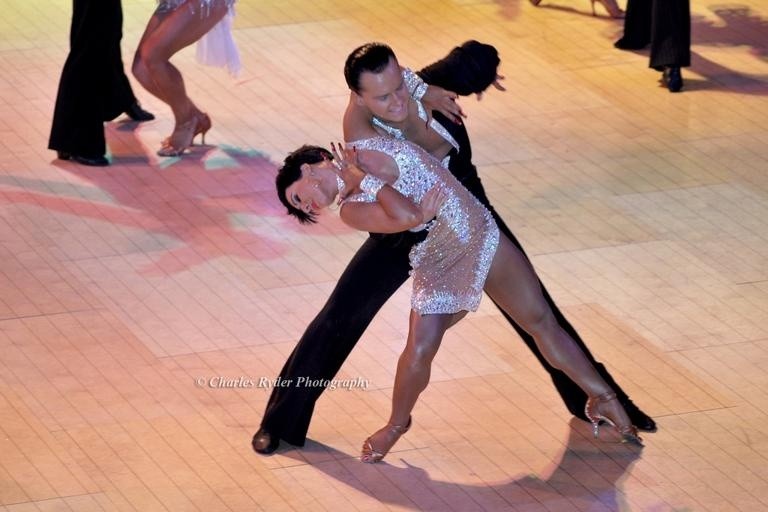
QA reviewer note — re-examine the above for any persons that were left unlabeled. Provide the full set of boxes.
[131,0,239,156]
[251,39,656,453]
[46,0,155,167]
[614,0,691,91]
[529,0,626,18]
[275,66,645,464]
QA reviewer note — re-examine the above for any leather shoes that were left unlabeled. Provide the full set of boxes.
[662,66,684,93]
[252,427,282,454]
[123,102,156,122]
[620,394,656,431]
[57,148,109,167]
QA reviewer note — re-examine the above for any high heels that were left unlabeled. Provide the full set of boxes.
[360,415,413,464]
[584,388,645,448]
[590,0,626,19]
[158,115,213,157]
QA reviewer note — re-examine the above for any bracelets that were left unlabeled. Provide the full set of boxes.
[400,68,429,100]
[359,174,387,200]
